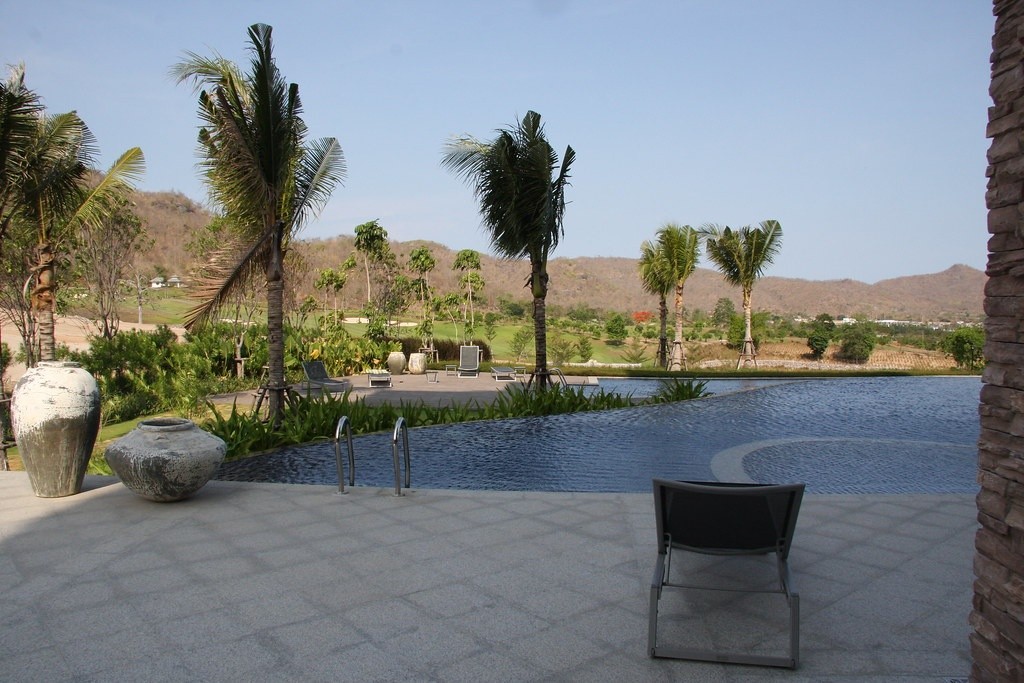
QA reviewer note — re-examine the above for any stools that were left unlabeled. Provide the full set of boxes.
[445,365,457,376]
[514,367,526,377]
[425,370,439,383]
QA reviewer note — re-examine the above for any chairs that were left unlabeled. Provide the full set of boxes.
[366,370,393,388]
[647,477,805,670]
[457,346,480,379]
[301,361,348,394]
[490,366,517,382]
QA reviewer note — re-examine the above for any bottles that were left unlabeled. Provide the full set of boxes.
[387,352,406,375]
[104,416,228,502]
[408,353,427,374]
[10,361,101,498]
